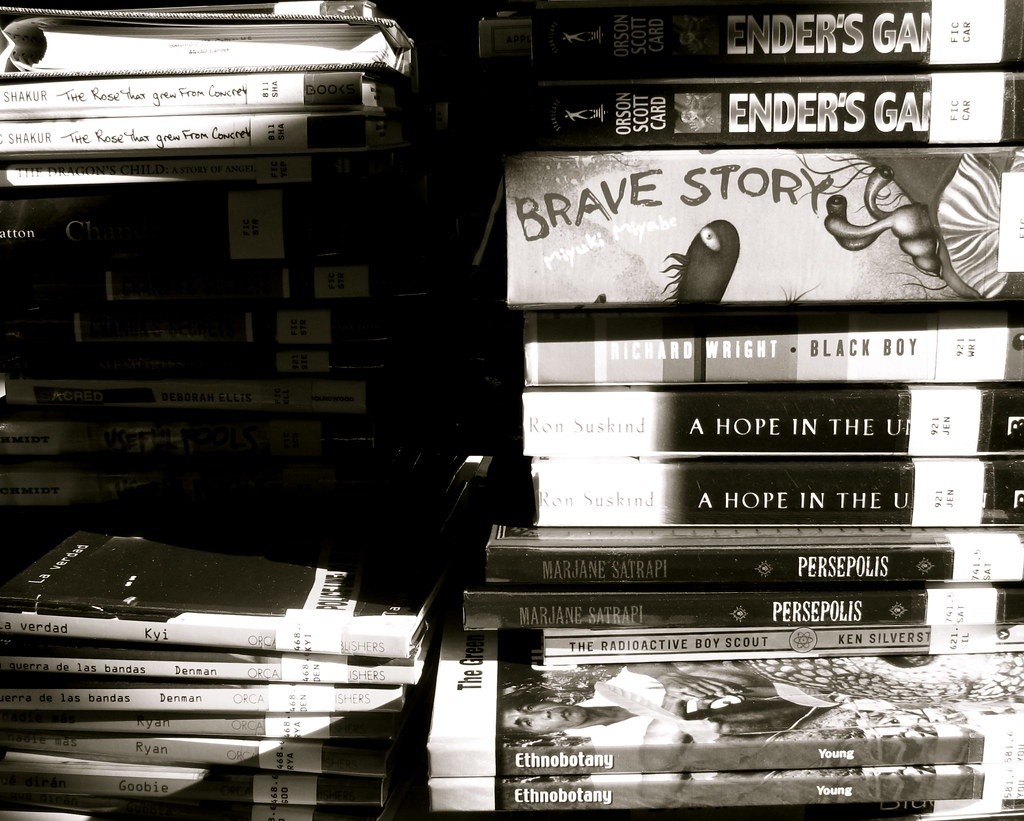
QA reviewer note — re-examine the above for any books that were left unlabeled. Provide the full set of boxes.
[0,0,1023,820]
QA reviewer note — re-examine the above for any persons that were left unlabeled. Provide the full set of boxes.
[499,662,776,767]
